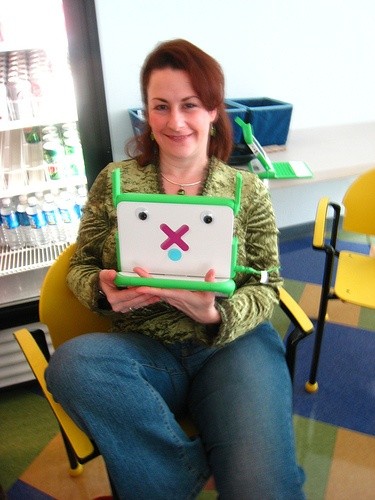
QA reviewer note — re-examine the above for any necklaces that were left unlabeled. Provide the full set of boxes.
[161,173,206,195]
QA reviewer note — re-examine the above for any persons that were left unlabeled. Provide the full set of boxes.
[42,37,309,500]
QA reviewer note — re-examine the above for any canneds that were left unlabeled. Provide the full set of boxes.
[0,50,56,121]
[23,127,40,143]
[40,122,84,179]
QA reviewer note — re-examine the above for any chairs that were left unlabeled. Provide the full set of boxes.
[13,242,315,477]
[305,166,375,394]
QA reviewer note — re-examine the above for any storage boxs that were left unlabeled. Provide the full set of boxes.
[127,99,246,155]
[228,97,293,146]
